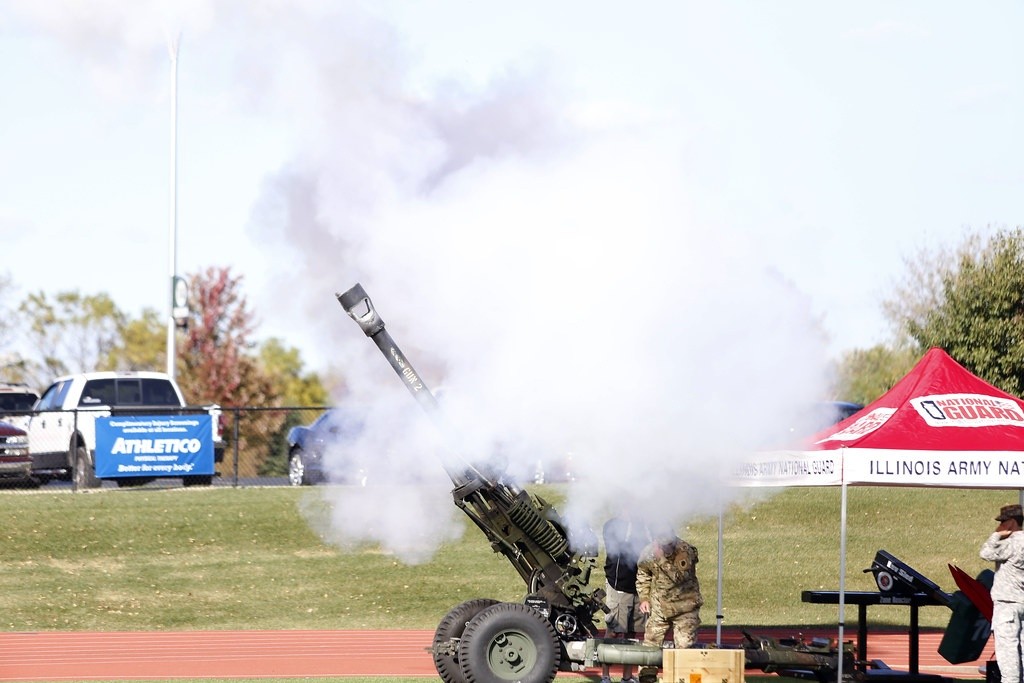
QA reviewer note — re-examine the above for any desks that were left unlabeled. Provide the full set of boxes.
[801,588,953,683]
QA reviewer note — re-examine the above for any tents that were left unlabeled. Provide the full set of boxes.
[718,346,1024,683]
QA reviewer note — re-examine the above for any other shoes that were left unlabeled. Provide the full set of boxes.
[621,675,639,683]
[601,676,611,683]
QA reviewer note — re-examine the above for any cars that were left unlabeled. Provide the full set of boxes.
[1,384,40,417]
[0,421,31,484]
[285,390,378,485]
[804,402,862,429]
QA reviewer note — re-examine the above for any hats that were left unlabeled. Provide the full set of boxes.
[995,504,1023,521]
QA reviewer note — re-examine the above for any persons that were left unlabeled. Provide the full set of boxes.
[635,520,704,683]
[602,499,651,683]
[978,504,1024,683]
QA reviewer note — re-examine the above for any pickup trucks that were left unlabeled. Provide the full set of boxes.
[3,371,227,491]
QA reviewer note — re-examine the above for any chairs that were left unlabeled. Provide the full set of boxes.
[143,383,169,405]
